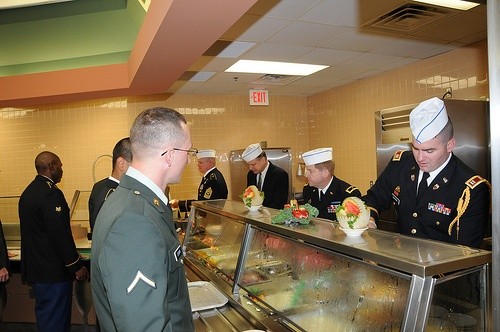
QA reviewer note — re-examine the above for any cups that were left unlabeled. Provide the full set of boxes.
[180,212,185,219]
[172,208,178,220]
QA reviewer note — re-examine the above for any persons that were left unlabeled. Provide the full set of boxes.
[172,149,229,216]
[86,136,131,239]
[300,147,361,222]
[0,219,18,312]
[240,143,290,209]
[89,108,198,332]
[359,97,492,300]
[18,151,86,332]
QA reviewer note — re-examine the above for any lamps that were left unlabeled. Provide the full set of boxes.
[296,162,306,175]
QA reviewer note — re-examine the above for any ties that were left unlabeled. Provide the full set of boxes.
[319,189,325,205]
[415,171,431,206]
[257,172,262,191]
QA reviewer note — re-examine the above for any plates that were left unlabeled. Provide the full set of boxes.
[361,295,407,303]
[423,305,476,331]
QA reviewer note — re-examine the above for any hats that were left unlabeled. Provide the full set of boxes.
[302,147,333,165]
[197,150,216,158]
[409,96,449,145]
[242,143,263,163]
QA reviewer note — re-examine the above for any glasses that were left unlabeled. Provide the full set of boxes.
[160,147,198,158]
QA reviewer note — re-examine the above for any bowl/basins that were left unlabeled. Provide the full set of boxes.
[206,226,221,235]
[339,226,368,237]
[244,205,262,211]
